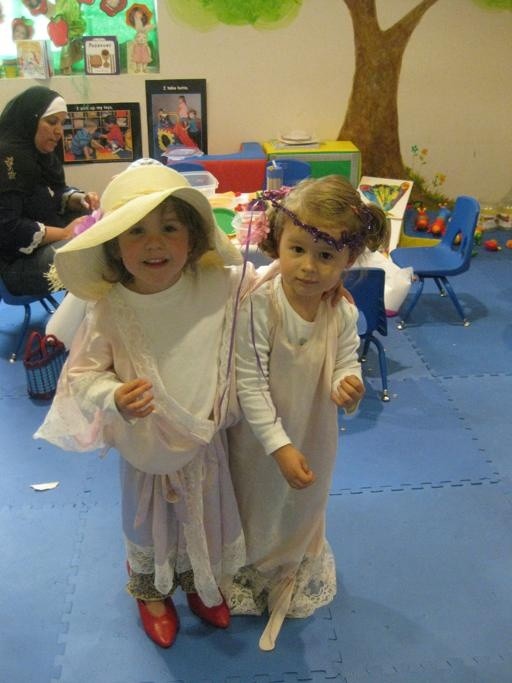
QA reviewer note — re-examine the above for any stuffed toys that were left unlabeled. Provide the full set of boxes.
[414,207,430,232]
[429,201,451,237]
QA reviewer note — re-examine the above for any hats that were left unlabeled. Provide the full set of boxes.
[53,163,245,303]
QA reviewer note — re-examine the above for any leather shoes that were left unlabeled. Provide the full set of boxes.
[137,596,179,648]
[187,586,231,629]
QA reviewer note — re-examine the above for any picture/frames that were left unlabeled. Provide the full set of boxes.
[57,101,140,165]
[82,35,120,76]
[145,77,209,156]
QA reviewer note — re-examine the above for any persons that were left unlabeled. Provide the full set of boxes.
[157,96,201,148]
[228,172,387,650]
[69,115,124,163]
[33,164,353,650]
[1,85,98,299]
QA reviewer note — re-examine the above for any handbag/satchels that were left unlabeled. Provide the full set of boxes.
[24,330,70,399]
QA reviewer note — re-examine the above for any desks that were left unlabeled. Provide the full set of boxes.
[93,181,414,357]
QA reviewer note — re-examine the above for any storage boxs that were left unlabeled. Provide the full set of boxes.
[178,170,219,199]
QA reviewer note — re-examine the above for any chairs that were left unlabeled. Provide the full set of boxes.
[3,282,68,363]
[324,262,394,404]
[389,194,480,329]
